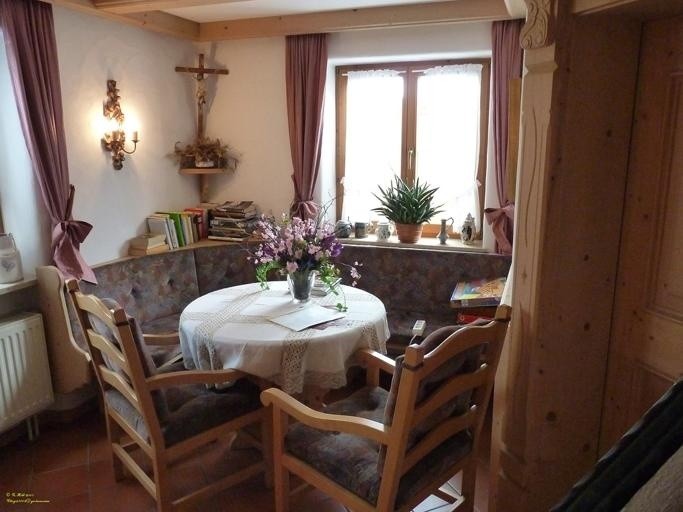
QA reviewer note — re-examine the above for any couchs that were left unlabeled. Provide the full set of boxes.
[63,238,516,377]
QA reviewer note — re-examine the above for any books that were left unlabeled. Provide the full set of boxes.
[128,201,259,257]
[287,274,343,296]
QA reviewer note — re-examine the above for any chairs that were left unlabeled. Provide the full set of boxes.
[258,304,513,512]
[66,278,274,509]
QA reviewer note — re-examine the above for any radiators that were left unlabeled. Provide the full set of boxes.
[0,311,55,440]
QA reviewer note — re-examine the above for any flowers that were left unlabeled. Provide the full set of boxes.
[245,193,365,315]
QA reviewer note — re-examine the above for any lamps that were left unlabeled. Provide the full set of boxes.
[101,118,142,172]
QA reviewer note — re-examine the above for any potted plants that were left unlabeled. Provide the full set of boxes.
[371,174,449,243]
[172,134,237,170]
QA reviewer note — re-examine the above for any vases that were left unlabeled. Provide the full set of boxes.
[284,266,316,303]
